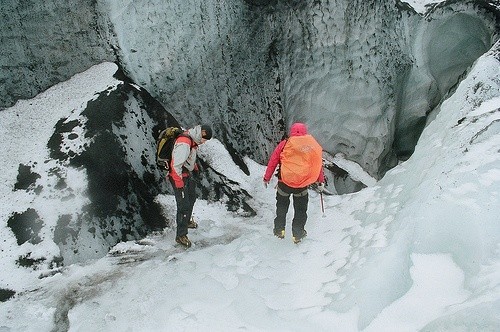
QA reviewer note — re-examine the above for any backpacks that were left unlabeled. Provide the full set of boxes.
[155,124,193,170]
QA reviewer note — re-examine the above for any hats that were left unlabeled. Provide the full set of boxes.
[201,124,212,140]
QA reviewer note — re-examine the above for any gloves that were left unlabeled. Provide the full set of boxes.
[178,187,185,194]
[264,181,270,188]
[317,183,324,192]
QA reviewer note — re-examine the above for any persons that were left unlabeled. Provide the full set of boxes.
[264,122,325,243]
[167,124,212,246]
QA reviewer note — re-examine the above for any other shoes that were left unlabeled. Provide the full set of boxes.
[274,228,285,239]
[293,230,307,244]
[188,221,197,228]
[175,235,191,247]
